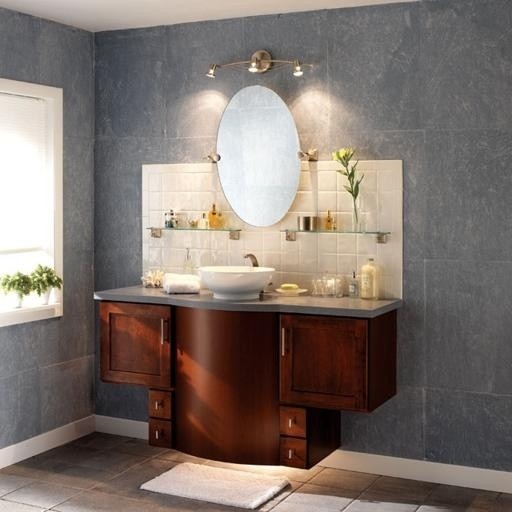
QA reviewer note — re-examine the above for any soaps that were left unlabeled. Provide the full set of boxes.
[280,283,299,291]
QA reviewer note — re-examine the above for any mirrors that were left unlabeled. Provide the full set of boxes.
[214,83,301,228]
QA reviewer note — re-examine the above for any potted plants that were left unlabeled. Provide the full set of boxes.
[28,264,64,308]
[0,269,31,308]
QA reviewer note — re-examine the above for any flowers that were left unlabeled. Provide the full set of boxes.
[332,146,366,224]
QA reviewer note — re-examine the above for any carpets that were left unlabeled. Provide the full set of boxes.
[138,462,289,509]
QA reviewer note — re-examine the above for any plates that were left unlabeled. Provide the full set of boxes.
[275,289,307,293]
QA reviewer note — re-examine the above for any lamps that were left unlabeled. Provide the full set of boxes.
[204,49,305,78]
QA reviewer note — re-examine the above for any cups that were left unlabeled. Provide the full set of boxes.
[312,277,345,296]
[297,216,317,231]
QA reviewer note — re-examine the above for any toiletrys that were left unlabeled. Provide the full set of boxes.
[163,204,226,230]
[360,257,386,300]
[295,209,338,232]
[348,270,358,296]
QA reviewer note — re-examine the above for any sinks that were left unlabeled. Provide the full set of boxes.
[197,265,275,301]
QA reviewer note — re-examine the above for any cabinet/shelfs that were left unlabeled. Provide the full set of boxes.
[147,387,175,450]
[100,302,173,387]
[277,407,343,470]
[280,313,398,415]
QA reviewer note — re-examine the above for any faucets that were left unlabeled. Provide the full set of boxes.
[244,252,258,267]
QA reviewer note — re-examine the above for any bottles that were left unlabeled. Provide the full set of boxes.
[348,272,359,296]
[162,205,228,230]
[324,209,337,230]
[361,258,381,300]
